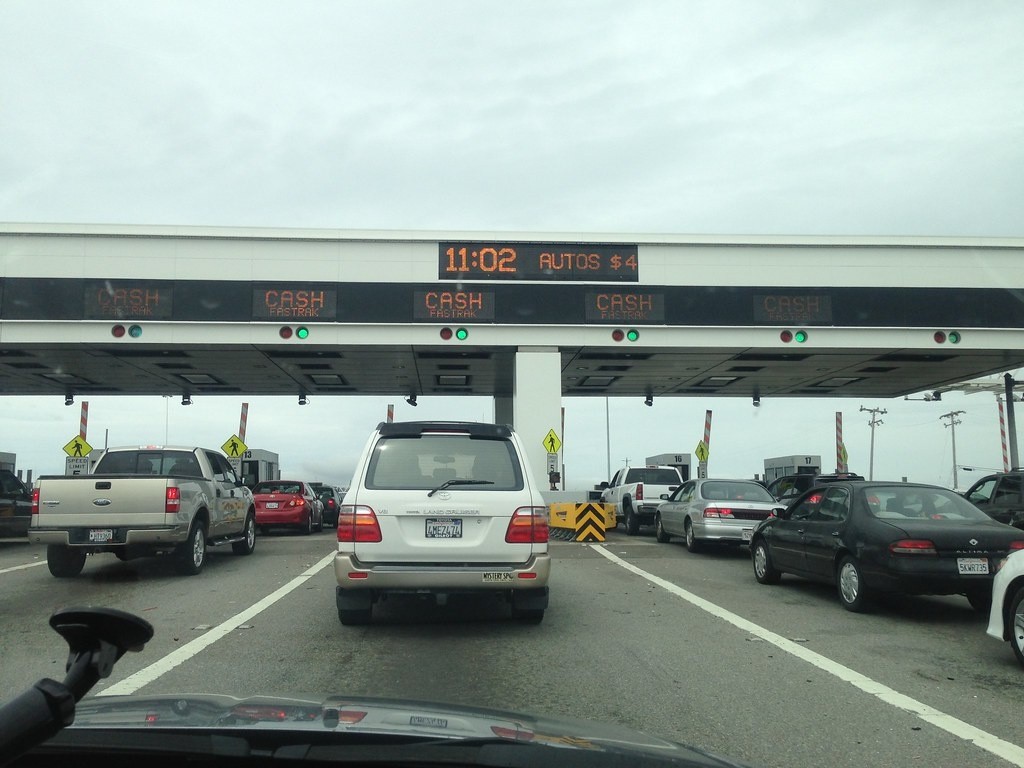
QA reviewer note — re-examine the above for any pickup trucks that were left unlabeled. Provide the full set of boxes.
[26,444,254,577]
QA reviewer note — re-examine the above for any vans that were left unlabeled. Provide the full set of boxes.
[334,419,552,626]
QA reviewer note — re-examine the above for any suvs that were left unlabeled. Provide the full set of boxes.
[598,465,685,536]
[962,465,1024,532]
[767,469,865,520]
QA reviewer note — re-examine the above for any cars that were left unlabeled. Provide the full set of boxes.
[654,476,789,554]
[249,480,325,533]
[747,479,1024,611]
[307,486,340,526]
[986,548,1024,666]
[0,469,31,539]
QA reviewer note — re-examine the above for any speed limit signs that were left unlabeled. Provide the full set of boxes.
[546,453,558,474]
[62,456,89,476]
[698,461,707,480]
[226,457,241,477]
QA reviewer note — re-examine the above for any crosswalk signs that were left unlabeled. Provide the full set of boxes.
[64,436,93,456]
[221,434,248,457]
[695,439,709,463]
[540,428,562,453]
